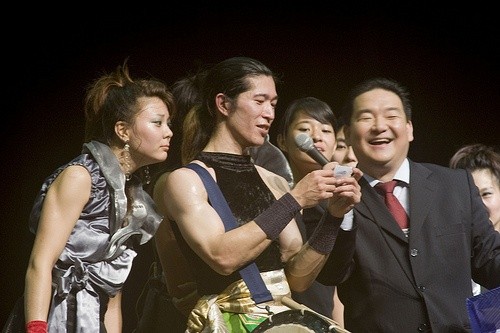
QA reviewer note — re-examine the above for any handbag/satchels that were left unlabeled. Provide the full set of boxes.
[135,262,186,333]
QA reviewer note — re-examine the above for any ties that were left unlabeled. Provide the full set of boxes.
[375,180,411,238]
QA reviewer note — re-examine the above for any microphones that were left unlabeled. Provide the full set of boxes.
[295,133,331,166]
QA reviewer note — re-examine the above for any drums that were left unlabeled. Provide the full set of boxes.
[251,309,351,333]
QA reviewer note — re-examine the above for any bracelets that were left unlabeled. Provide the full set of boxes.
[25,321,48,333]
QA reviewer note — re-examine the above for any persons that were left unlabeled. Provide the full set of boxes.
[152,55,363,333]
[305,77,500,333]
[0,75,174,333]
[138,95,499,333]
